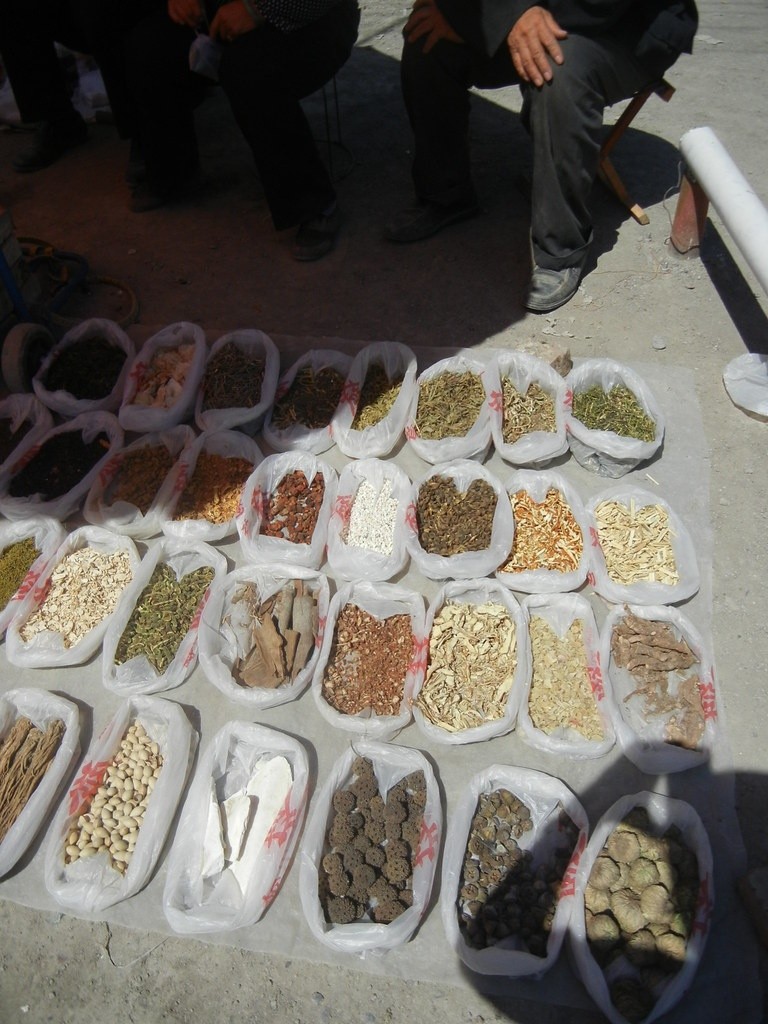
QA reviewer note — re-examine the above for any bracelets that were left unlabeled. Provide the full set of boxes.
[243,0,266,24]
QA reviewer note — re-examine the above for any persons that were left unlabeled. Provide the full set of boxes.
[0,0,361,260]
[378,0,700,311]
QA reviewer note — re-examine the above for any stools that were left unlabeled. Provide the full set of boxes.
[299,53,355,186]
[587,80,676,225]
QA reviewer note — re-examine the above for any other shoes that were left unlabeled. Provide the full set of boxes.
[11,111,88,171]
[128,173,200,211]
[292,206,345,260]
[126,141,159,187]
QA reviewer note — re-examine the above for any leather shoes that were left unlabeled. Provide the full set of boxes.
[521,244,591,311]
[383,192,480,241]
